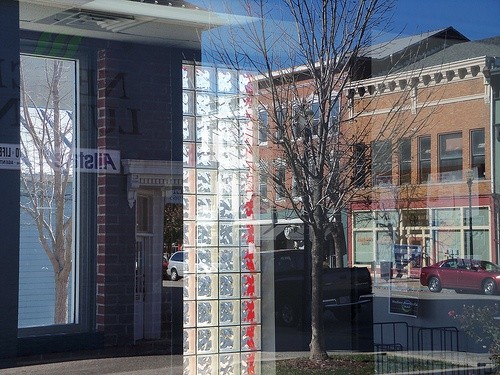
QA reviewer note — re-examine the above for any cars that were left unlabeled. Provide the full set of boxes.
[420,258,500,295]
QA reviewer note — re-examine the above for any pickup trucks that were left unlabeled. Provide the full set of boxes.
[257,248,373,327]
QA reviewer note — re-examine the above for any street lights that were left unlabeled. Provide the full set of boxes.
[465,167,475,259]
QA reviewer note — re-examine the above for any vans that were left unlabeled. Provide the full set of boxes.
[166,250,230,281]
